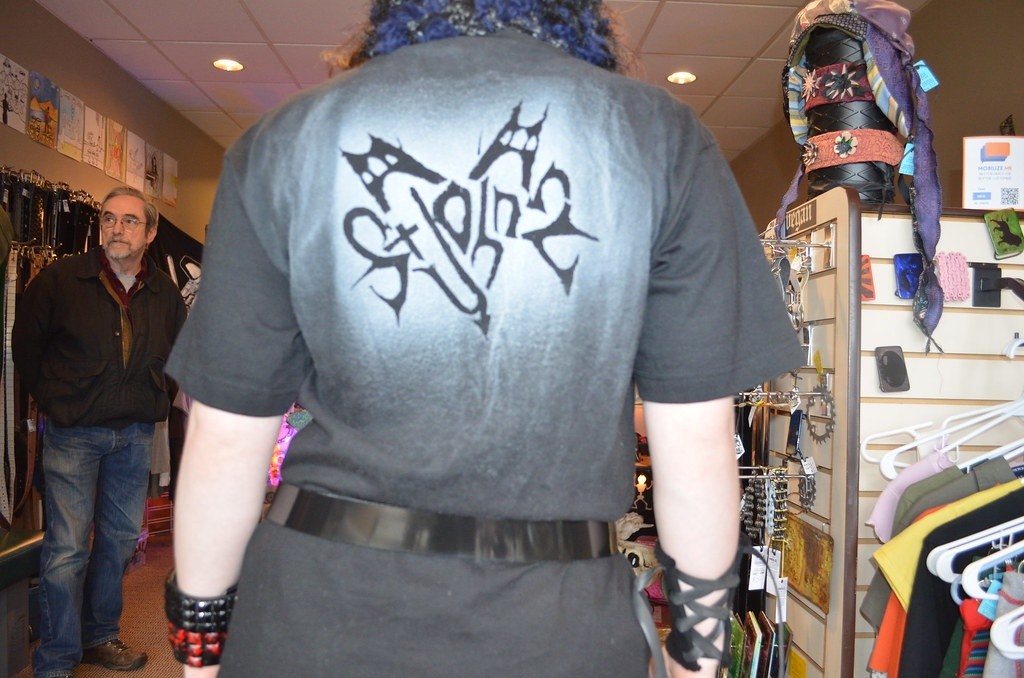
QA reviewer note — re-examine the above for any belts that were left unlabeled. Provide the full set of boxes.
[0,164,104,533]
[268,478,619,562]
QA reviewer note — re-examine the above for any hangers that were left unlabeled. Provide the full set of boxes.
[859,336,1024,661]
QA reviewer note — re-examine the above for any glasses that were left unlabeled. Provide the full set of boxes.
[98,214,151,232]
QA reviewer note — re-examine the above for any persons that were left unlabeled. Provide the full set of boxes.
[162,0,807,678]
[12,187,188,678]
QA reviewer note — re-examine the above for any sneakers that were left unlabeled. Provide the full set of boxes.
[81,640,149,670]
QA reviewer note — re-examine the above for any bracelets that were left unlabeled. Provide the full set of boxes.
[163,568,238,667]
